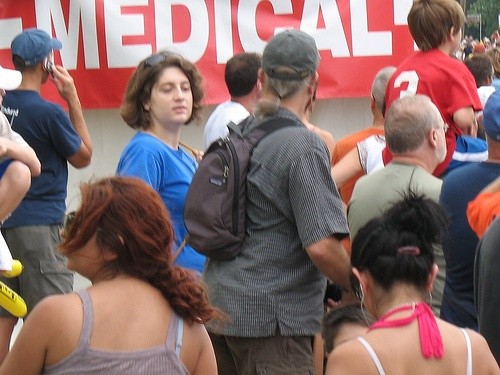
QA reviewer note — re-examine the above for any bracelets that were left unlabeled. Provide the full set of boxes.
[342,287,353,294]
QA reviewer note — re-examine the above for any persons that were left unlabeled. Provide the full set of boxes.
[199,29,361,375]
[0,0,500,375]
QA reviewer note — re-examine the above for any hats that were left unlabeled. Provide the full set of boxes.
[483,90,500,142]
[0,64,22,91]
[10,29,62,71]
[262,29,320,80]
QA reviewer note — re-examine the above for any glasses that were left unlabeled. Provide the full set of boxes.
[143,53,167,71]
[64,213,76,233]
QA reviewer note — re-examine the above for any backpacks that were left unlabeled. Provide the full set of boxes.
[183,117,303,261]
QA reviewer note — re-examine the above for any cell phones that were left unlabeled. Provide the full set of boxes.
[46,61,54,73]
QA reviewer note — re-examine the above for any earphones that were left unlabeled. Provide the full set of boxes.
[360,283,363,293]
